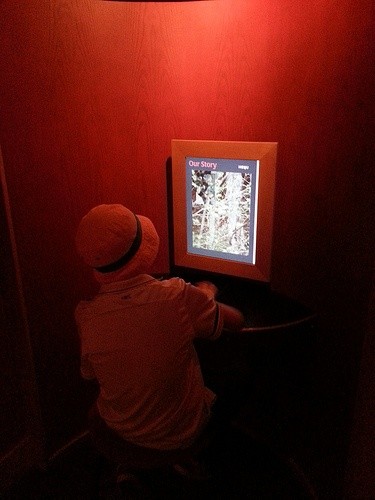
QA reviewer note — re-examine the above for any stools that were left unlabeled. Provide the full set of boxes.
[78,399,204,500]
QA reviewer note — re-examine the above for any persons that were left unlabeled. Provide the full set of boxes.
[75,204,242,449]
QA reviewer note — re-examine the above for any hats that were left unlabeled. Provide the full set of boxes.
[76,204,160,285]
